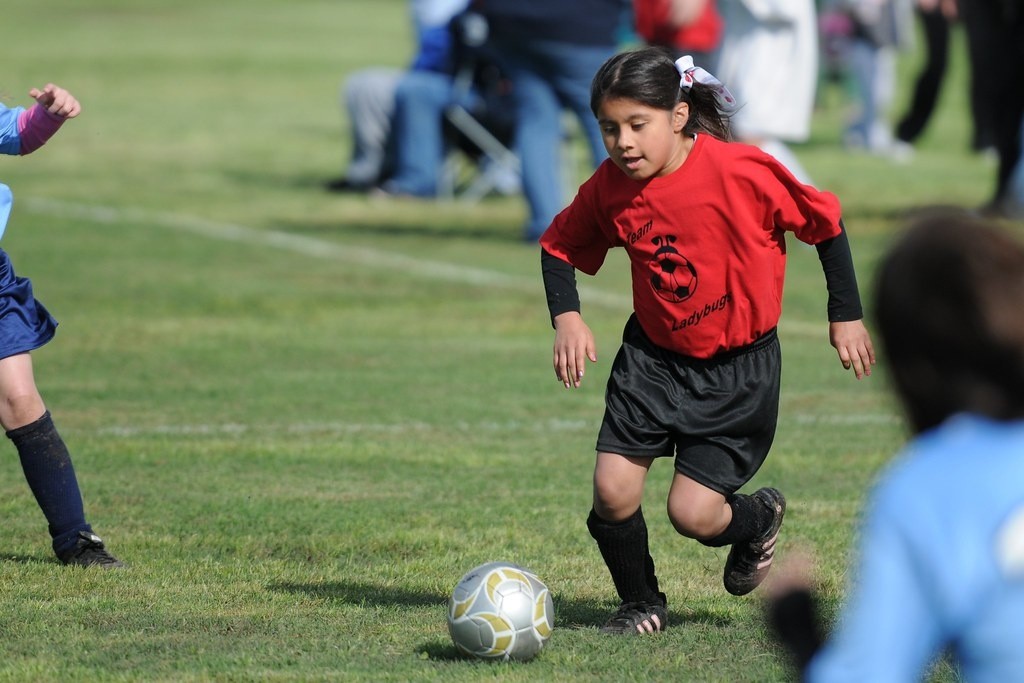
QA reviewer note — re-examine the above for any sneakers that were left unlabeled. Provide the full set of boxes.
[598,598,666,637]
[723,487,786,597]
[54,528,124,572]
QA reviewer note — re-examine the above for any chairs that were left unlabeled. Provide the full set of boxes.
[435,100,523,218]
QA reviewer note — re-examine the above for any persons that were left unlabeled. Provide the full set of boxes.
[540,48,876,636]
[335,0,1024,242]
[0,85,121,566]
[765,207,1024,683]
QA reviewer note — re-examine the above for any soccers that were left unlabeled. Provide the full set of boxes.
[444,558,555,665]
